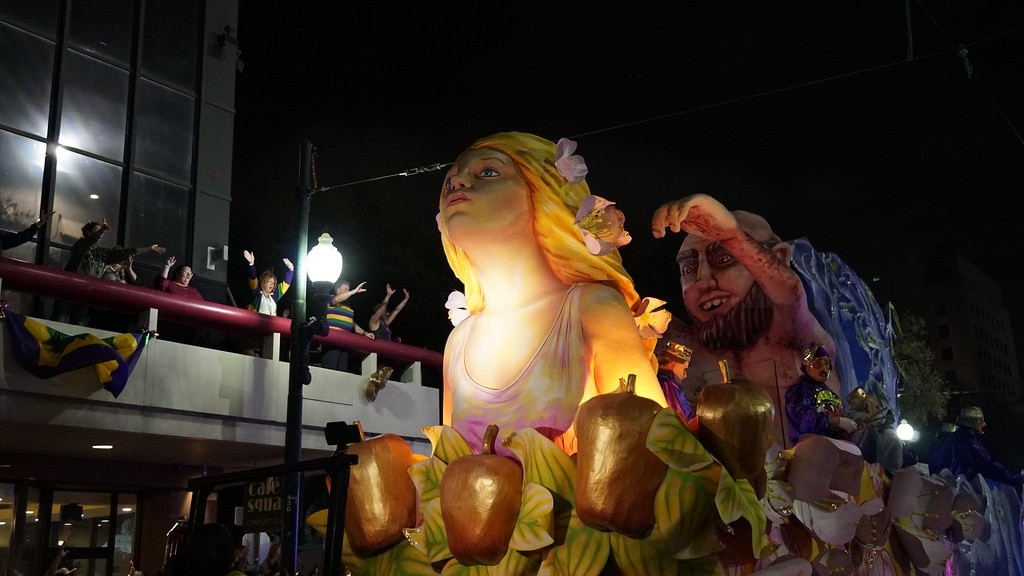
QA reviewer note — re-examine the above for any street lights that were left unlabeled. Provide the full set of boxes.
[283,228,345,576]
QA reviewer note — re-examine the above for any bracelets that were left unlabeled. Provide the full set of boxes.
[361,329,365,335]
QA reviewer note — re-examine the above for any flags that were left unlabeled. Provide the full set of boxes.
[4,309,150,399]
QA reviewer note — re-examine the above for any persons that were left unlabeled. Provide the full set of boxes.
[436,131,669,458]
[369,282,409,380]
[323,279,375,371]
[0,209,56,251]
[653,339,694,421]
[183,522,248,576]
[783,342,867,446]
[651,193,841,453]
[935,405,1024,486]
[243,250,294,358]
[260,544,284,576]
[54,217,210,346]
